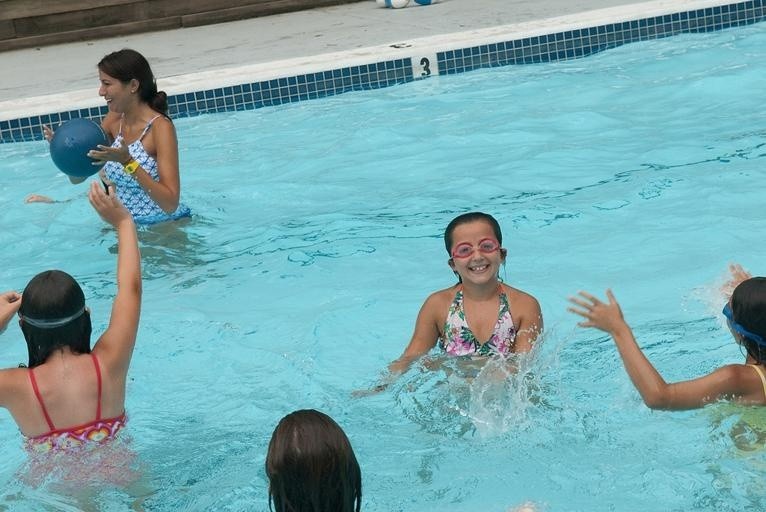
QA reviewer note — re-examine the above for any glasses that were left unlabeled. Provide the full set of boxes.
[722,303,765,347]
[453,238,499,259]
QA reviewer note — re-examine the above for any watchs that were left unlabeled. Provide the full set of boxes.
[125,160,140,174]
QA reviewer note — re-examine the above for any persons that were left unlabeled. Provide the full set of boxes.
[23,191,88,203]
[1,180,144,494]
[563,260,766,454]
[41,47,192,226]
[347,210,545,399]
[263,407,363,512]
